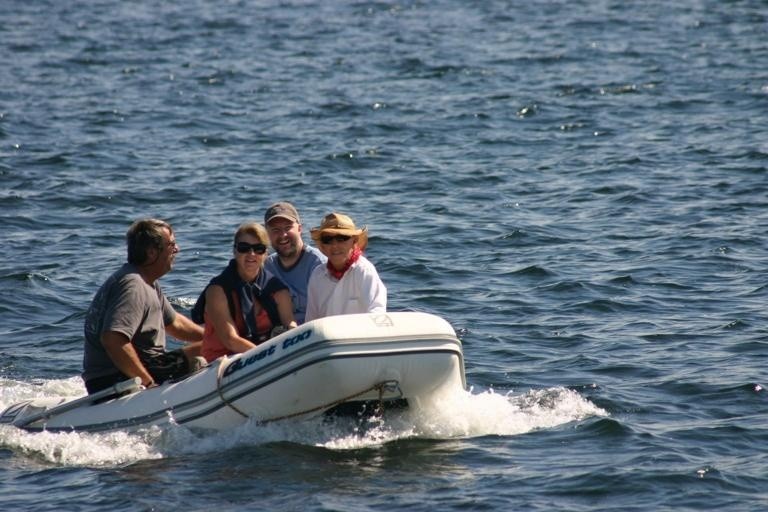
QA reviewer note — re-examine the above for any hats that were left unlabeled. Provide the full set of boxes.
[265,203,299,224]
[312,214,368,256]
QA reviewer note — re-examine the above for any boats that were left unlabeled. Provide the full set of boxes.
[0,312,466,438]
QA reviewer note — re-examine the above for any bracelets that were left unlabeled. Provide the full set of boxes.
[145,380,155,387]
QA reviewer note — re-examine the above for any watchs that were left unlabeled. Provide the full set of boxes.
[301,212,386,322]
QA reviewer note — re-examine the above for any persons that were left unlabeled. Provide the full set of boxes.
[83,219,209,405]
[263,199,327,322]
[202,221,299,362]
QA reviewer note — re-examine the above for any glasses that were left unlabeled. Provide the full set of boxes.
[235,242,267,255]
[320,235,350,244]
